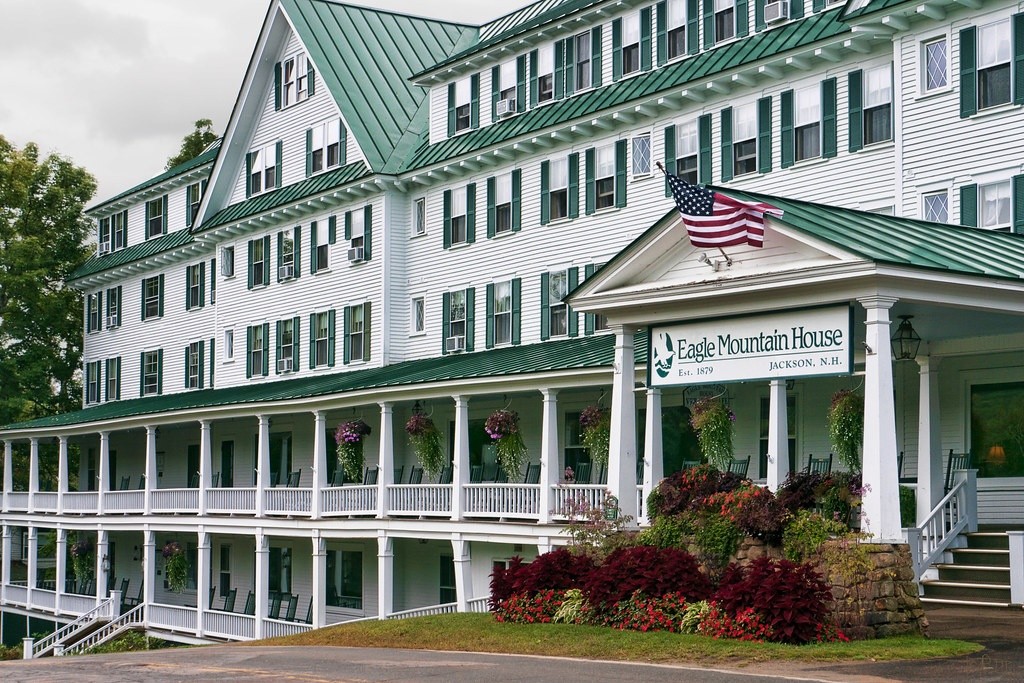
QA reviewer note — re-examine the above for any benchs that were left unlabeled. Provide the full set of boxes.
[35,448,970,624]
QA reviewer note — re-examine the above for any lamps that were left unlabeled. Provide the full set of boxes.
[894,315,920,360]
[419,539,429,544]
[786,380,794,390]
[412,400,423,415]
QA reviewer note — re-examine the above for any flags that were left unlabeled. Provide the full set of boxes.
[666,170,784,248]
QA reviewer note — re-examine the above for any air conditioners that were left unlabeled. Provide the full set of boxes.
[99,241,109,253]
[446,335,465,353]
[279,265,293,279]
[347,247,363,261]
[497,98,513,115]
[105,315,116,327]
[277,358,292,372]
[764,1,787,23]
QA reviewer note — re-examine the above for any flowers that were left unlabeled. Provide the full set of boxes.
[71,541,94,584]
[690,396,736,470]
[407,414,447,480]
[830,389,865,478]
[162,541,190,596]
[583,403,613,466]
[335,417,372,484]
[484,408,531,483]
